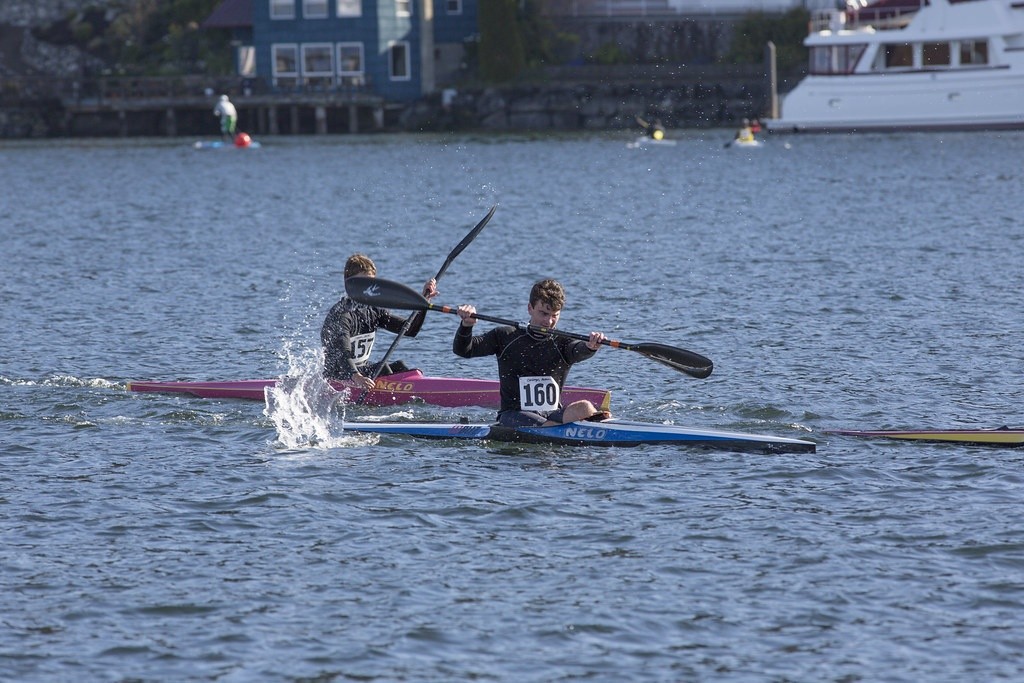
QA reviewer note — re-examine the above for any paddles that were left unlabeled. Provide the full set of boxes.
[633,115,649,130]
[344,276,714,379]
[355,203,499,407]
[723,133,738,148]
[204,88,243,138]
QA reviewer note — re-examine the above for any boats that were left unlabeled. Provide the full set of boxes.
[638,137,676,147]
[843,427,1024,446]
[342,419,820,455]
[765,0,1024,130]
[192,140,260,148]
[727,138,761,149]
[115,369,612,416]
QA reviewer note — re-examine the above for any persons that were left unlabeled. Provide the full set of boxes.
[321,255,439,390]
[735,118,760,142]
[453,279,606,426]
[214,94,237,142]
[646,119,666,139]
